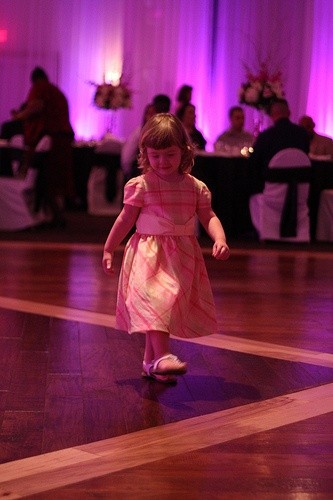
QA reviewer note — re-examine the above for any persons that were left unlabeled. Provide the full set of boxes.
[120,85,332,203]
[102,113,231,386]
[7,67,86,229]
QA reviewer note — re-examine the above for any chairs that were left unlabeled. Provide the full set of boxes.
[0,134,124,232]
[249,148,333,242]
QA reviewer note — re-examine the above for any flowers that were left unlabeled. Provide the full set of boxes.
[86,73,133,109]
[238,40,287,110]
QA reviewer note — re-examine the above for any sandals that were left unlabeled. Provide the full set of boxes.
[141,360,177,383]
[152,353,187,374]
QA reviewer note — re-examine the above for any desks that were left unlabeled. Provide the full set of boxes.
[191,150,330,242]
